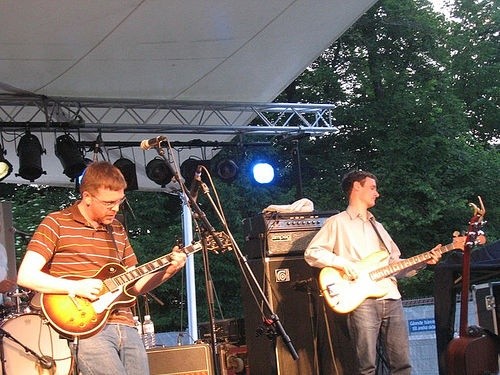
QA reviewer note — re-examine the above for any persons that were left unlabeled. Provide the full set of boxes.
[0,244,15,310]
[15,161,188,375]
[305,171,442,375]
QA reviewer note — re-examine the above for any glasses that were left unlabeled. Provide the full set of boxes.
[92,195,126,207]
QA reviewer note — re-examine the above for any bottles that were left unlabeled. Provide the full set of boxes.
[133,315,143,344]
[143,315,156,350]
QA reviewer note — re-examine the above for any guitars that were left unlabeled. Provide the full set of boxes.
[444,195,500,375]
[318,231,486,315]
[39,231,234,345]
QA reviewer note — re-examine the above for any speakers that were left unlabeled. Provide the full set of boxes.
[145,343,215,375]
[240,256,320,374]
[307,261,364,375]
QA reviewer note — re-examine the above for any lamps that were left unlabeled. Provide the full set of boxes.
[0,121,13,182]
[49,122,92,182]
[179,141,277,188]
[15,121,47,182]
[112,142,138,191]
[143,147,174,188]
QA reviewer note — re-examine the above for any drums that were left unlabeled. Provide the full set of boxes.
[0,312,74,375]
[25,287,41,309]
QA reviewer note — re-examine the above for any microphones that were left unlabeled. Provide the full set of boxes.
[190,166,202,202]
[291,277,314,288]
[140,135,165,150]
[39,355,53,369]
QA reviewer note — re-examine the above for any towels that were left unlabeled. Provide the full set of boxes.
[261,197,315,214]
